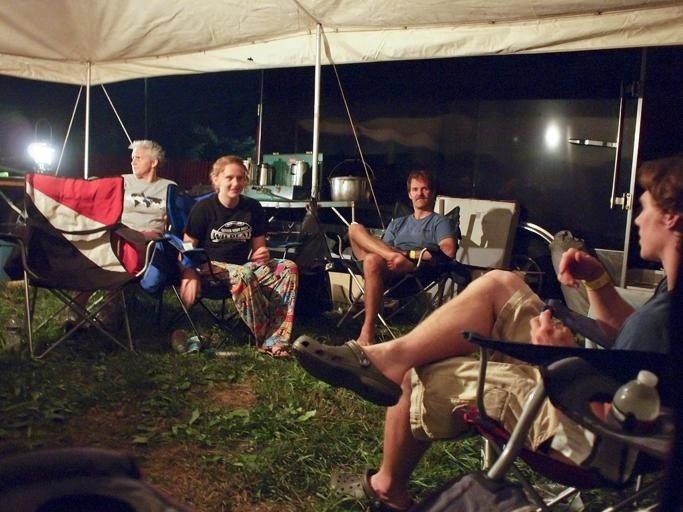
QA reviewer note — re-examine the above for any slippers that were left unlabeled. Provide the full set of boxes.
[292,335,403,409]
[330,467,417,512]
[258,344,290,359]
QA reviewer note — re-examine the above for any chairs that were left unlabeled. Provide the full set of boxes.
[1,172,683,512]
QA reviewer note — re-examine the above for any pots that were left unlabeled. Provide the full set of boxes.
[328,175,376,201]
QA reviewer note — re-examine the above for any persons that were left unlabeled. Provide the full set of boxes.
[59,139,182,344]
[290,154,682,510]
[166,154,300,359]
[346,168,459,345]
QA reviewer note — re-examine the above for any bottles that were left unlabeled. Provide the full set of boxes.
[592,370,660,487]
[171,329,187,353]
[290,160,309,187]
[258,164,275,185]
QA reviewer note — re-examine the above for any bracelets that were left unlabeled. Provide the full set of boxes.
[579,271,611,292]
[408,250,416,260]
